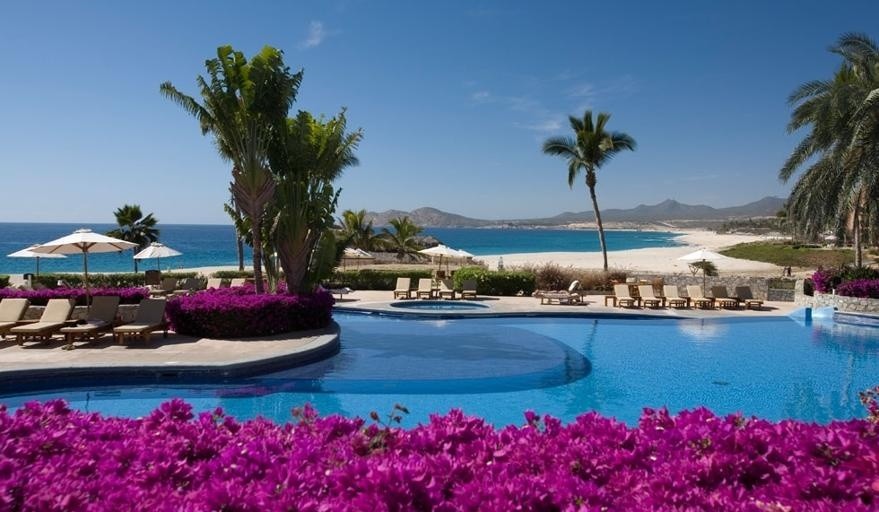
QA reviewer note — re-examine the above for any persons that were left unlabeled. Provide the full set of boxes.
[497,256,502,269]
[178,264,180,269]
[181,265,183,269]
[22,273,35,287]
[785,264,792,276]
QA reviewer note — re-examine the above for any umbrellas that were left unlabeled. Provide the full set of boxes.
[681,249,731,297]
[6,243,68,283]
[26,228,141,313]
[417,243,454,298]
[444,248,466,276]
[456,249,475,267]
[134,242,183,271]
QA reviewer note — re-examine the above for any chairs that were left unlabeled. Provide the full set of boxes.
[532,280,584,305]
[327,286,350,301]
[393,277,411,300]
[613,284,763,310]
[417,278,432,300]
[0,278,248,350]
[439,280,455,300]
[461,279,477,299]
